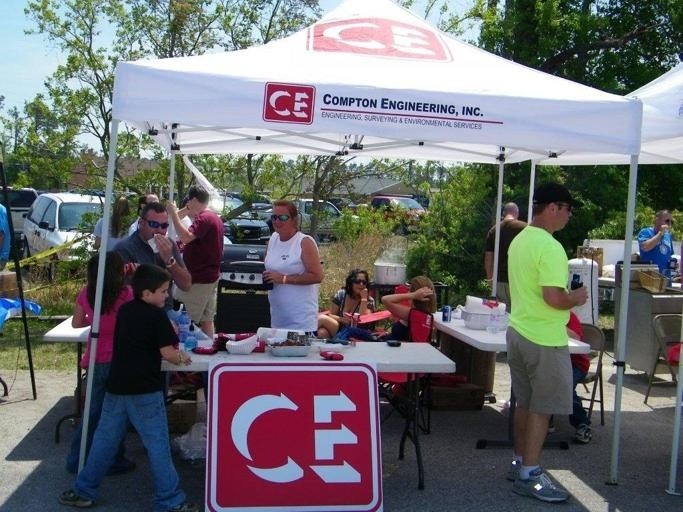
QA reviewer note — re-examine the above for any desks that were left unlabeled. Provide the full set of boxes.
[43,313,91,444]
[161,332,457,488]
[433,316,591,438]
[0,308,22,396]
[597,285,615,315]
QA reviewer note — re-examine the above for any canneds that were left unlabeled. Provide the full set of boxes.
[573,273,580,282]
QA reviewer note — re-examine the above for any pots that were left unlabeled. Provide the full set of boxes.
[373,262,407,286]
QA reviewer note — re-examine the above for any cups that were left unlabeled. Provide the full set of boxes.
[668,258,677,269]
[350,312,361,329]
[262,274,274,291]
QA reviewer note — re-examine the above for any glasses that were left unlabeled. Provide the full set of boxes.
[269,214,291,222]
[142,218,169,230]
[352,278,367,285]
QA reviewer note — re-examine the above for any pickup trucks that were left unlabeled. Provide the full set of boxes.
[225,189,431,242]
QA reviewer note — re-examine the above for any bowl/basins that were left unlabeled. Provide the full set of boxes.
[459,307,511,333]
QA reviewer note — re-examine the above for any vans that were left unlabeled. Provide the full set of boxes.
[1,186,39,239]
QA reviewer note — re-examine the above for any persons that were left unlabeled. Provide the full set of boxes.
[2,183,681,510]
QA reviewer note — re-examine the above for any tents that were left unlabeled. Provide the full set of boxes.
[79,1,683,476]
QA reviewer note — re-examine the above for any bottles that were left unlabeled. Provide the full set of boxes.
[175,304,198,353]
[570,273,583,290]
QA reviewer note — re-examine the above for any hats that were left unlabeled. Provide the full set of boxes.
[531,183,584,207]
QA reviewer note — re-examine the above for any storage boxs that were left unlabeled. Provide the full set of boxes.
[577,245,603,276]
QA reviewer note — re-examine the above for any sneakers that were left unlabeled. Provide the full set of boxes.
[548,414,555,433]
[107,457,136,477]
[506,459,569,503]
[169,500,201,512]
[574,423,592,445]
[56,489,96,508]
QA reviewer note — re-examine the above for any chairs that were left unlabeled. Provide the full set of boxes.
[382,328,443,434]
[164,385,204,405]
[578,323,606,425]
[644,313,683,403]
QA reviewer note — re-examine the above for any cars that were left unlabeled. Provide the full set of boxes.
[179,195,270,244]
[21,191,107,283]
